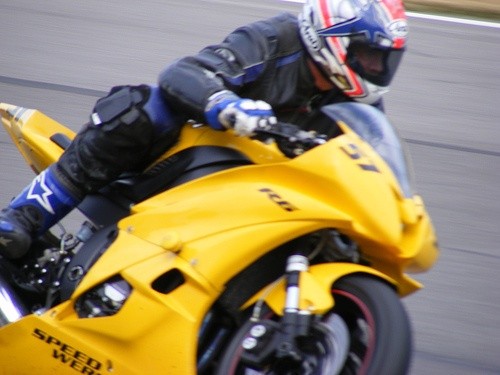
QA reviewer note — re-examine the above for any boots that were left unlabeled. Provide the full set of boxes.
[1,162,87,261]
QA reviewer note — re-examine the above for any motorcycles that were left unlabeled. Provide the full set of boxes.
[0,101,440,375]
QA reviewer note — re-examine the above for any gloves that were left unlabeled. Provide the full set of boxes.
[202,89,277,138]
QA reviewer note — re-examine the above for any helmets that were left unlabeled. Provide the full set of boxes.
[298,0,408,106]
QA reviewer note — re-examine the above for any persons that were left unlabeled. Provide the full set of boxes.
[0,0,408,262]
